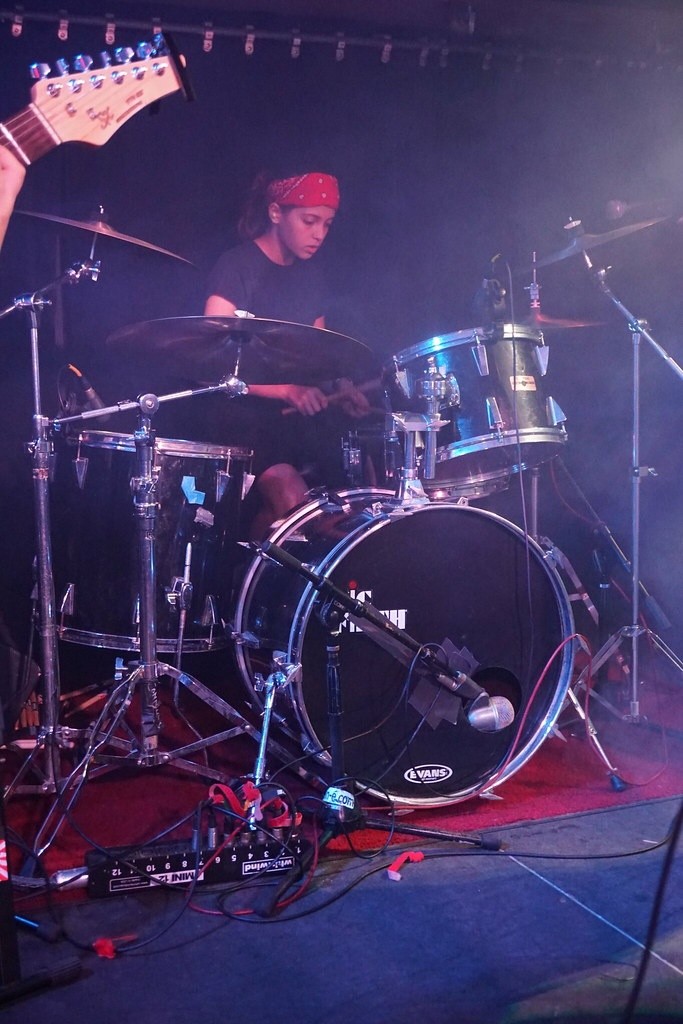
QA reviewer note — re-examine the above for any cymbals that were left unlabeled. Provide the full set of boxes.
[514,306,612,330]
[10,202,201,269]
[100,315,372,391]
[505,213,674,280]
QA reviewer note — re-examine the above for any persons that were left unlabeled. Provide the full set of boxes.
[203,154,371,541]
[0,145,27,251]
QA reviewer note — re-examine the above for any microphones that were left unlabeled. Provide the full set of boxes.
[606,198,667,221]
[480,253,505,313]
[437,675,516,731]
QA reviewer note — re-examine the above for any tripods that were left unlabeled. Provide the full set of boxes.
[0,234,683,917]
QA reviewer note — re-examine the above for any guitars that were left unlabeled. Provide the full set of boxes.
[1,33,192,180]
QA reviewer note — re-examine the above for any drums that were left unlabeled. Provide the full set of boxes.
[38,321,583,808]
